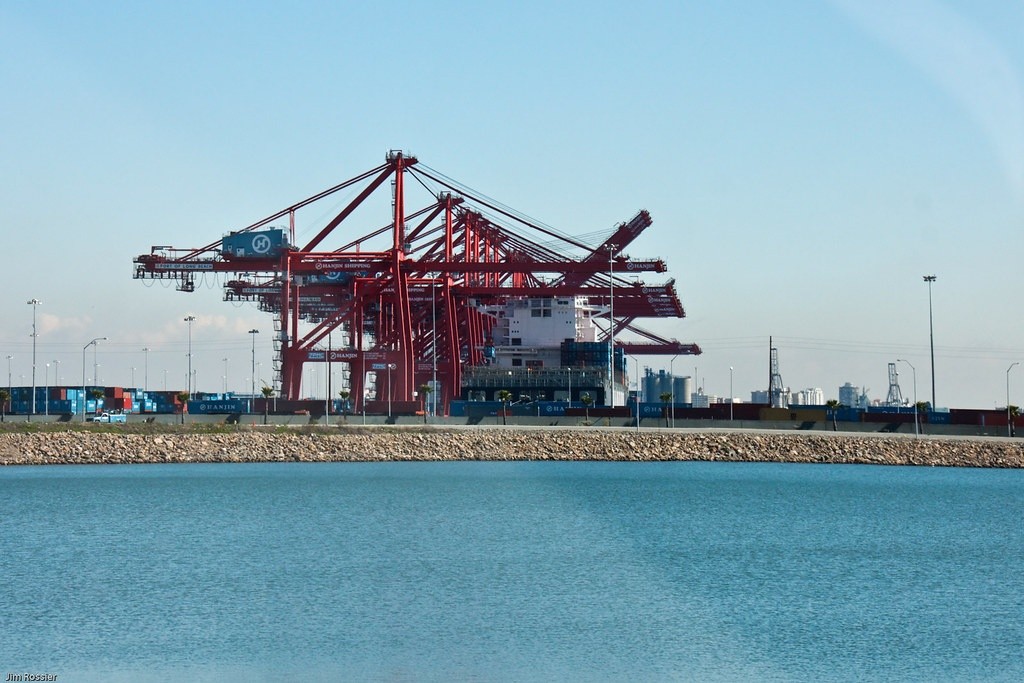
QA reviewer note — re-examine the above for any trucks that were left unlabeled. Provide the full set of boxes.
[85,412,128,425]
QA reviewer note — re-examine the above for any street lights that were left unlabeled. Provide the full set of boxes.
[923,274,937,413]
[670,349,691,429]
[6,353,14,415]
[1006,362,1020,437]
[45,363,50,418]
[567,367,573,409]
[53,359,60,385]
[605,243,621,409]
[81,337,109,423]
[184,314,196,401]
[27,298,42,413]
[729,366,735,423]
[615,350,640,435]
[896,358,920,440]
[248,328,259,423]
[142,347,150,392]
